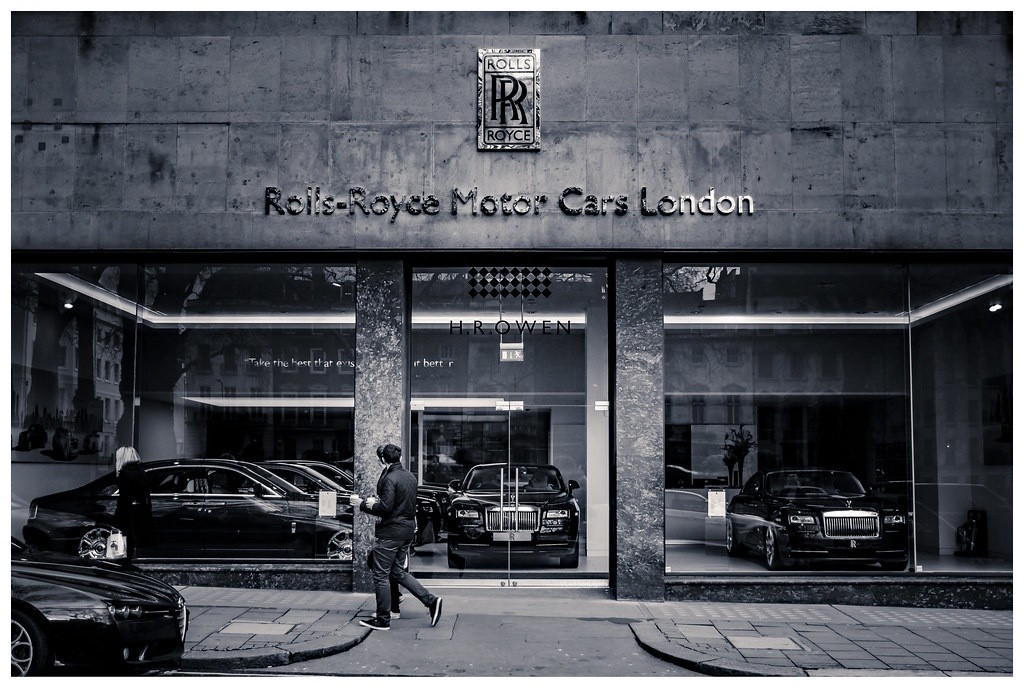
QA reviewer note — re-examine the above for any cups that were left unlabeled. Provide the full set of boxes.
[351,495,359,498]
[367,497,375,503]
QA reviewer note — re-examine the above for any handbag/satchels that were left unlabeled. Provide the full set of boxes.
[106,529,127,560]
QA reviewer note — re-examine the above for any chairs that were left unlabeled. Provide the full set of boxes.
[176,472,197,491]
[524,474,553,491]
[478,474,500,487]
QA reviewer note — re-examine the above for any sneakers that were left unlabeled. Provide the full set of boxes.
[429,597,442,627]
[372,611,400,620]
[359,616,391,630]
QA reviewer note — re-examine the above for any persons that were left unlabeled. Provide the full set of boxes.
[349,443,442,632]
[108,445,155,565]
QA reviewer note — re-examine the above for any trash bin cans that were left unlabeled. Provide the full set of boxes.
[967,510,988,558]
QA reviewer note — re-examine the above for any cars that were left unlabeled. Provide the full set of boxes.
[11,535,191,677]
[726,467,909,570]
[203,462,354,527]
[283,460,443,547]
[444,463,580,569]
[418,485,448,491]
[661,489,727,545]
[416,489,451,528]
[21,459,356,564]
